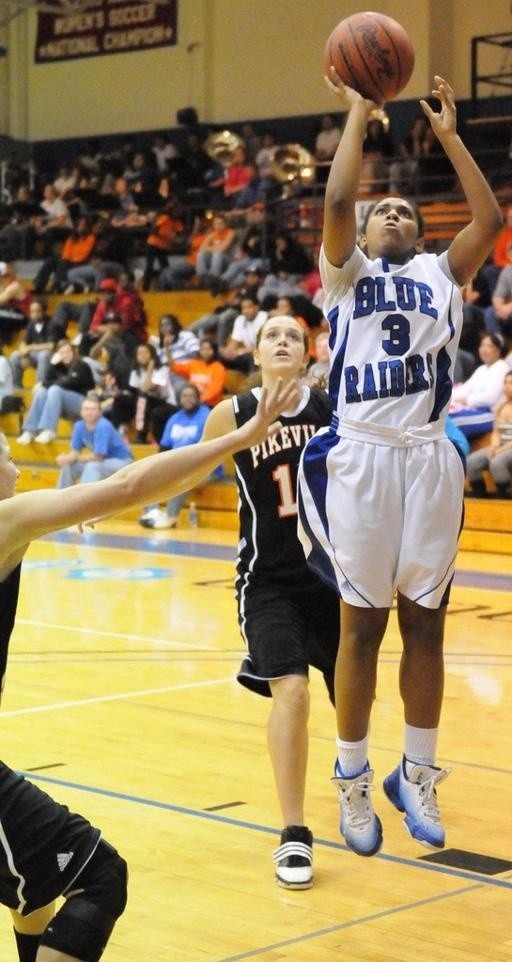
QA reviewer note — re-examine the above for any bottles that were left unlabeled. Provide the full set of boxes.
[190,500,198,530]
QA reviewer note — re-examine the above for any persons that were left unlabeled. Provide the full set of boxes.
[444,333,512,454]
[358,113,453,195]
[57,398,135,489]
[297,65,502,856]
[0,114,343,446]
[0,377,299,961]
[140,386,222,529]
[453,204,512,384]
[198,315,332,890]
[466,372,512,497]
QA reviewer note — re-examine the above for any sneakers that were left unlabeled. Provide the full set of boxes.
[17,432,36,444]
[272,825,313,890]
[334,759,382,856]
[384,752,444,850]
[139,507,177,528]
[36,431,57,443]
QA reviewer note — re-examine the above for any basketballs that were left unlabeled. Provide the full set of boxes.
[324,11,414,103]
